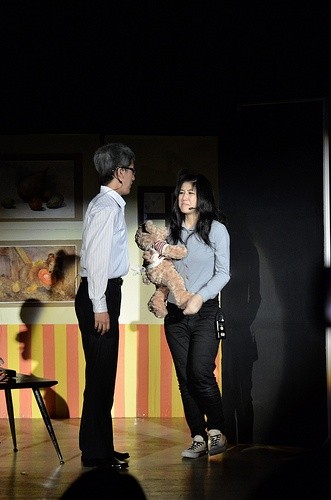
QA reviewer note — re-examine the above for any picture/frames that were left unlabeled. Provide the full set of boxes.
[0,153,83,222]
[0,245,77,304]
[137,186,171,230]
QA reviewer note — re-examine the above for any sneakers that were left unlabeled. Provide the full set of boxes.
[181,435,208,459]
[207,429,229,456]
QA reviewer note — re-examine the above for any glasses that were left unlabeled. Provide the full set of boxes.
[119,167,136,174]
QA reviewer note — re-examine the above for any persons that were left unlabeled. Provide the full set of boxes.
[75,143,135,471]
[164,172,234,459]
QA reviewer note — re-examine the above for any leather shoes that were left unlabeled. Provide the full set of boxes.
[80,450,128,470]
[112,451,130,460]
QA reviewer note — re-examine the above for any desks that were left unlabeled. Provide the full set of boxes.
[0,374,64,465]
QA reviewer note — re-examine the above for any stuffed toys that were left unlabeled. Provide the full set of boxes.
[135,221,194,318]
